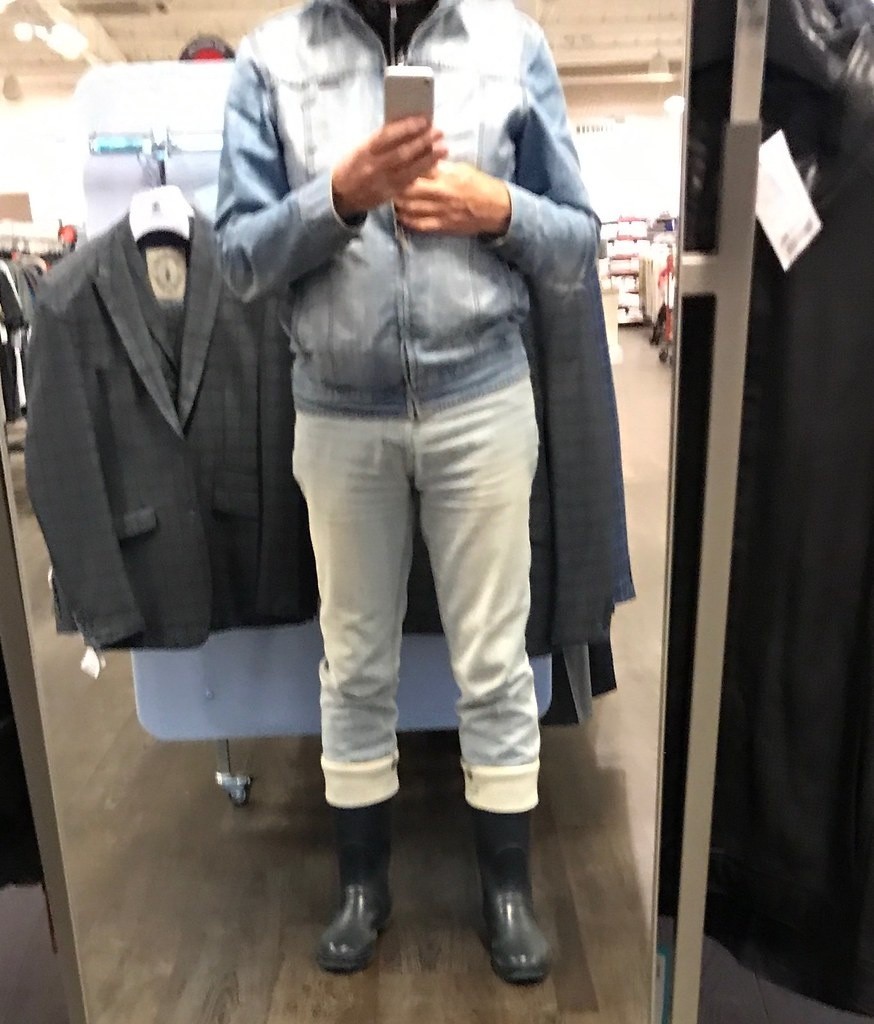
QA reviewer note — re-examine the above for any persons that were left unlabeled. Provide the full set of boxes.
[219,0,597,987]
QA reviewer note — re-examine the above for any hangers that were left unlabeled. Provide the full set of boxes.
[127,136,192,242]
[6,234,33,255]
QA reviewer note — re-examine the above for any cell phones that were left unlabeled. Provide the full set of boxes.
[386,68,433,157]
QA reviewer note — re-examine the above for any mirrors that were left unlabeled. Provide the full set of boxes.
[0,0,779,1024]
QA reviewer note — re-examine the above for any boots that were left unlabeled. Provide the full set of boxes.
[315,799,392,971]
[469,807,551,980]
